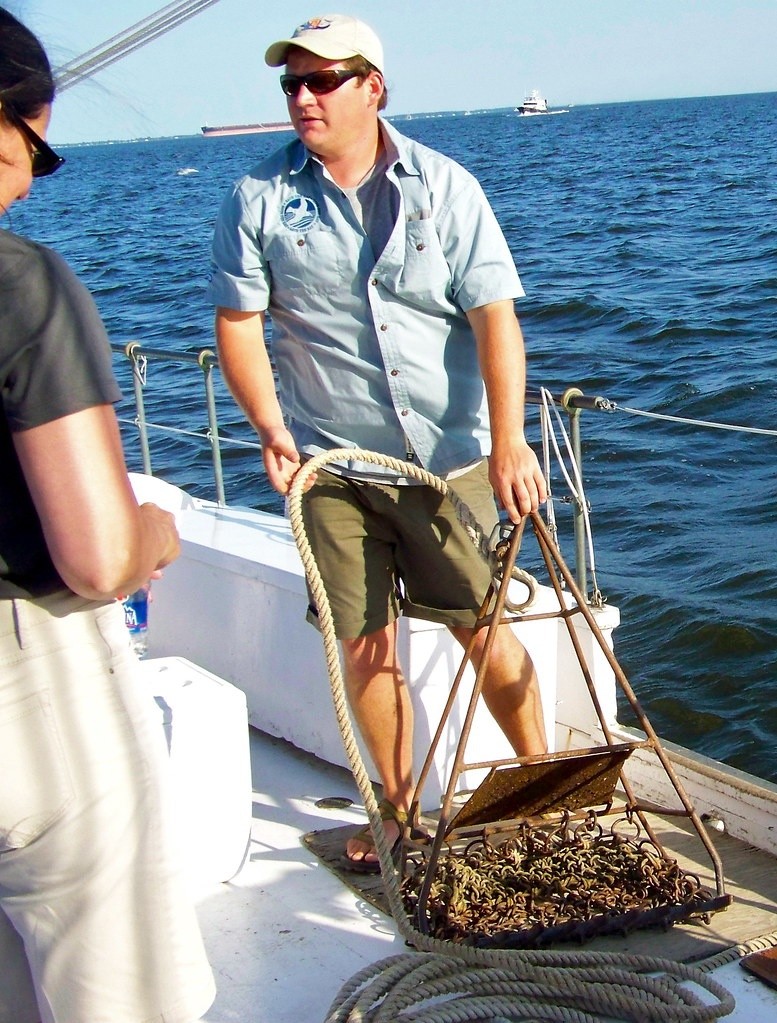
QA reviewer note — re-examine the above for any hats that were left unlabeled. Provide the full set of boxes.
[264,14,383,76]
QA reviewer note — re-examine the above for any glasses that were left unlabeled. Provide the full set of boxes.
[280,70,370,97]
[5,102,65,178]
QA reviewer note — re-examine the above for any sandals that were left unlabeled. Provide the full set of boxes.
[341,798,422,874]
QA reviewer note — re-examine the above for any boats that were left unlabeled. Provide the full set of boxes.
[517,88,549,114]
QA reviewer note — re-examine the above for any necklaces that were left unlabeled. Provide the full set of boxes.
[358,147,388,190]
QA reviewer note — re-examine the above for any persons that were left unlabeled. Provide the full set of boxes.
[210,12,552,867]
[0,7,219,1023]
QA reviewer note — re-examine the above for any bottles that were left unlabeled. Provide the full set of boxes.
[122,588,149,657]
